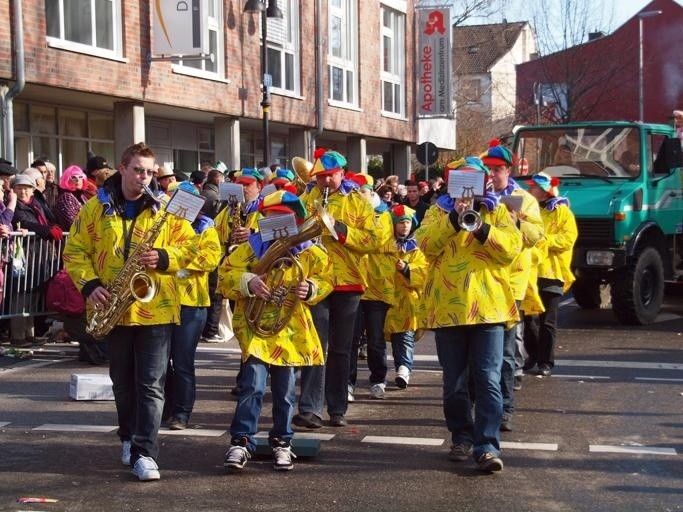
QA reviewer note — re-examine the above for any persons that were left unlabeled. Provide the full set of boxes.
[618,133,641,178]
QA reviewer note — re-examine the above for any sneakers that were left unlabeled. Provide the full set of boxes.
[129,455,161,481]
[447,441,475,462]
[522,355,552,376]
[121,440,132,466]
[268,436,295,471]
[11,336,49,349]
[347,384,356,402]
[358,343,368,358]
[512,375,522,390]
[329,414,347,427]
[368,382,386,400]
[394,364,411,390]
[223,436,252,470]
[469,447,504,472]
[159,415,189,430]
[230,386,242,397]
[292,412,324,429]
[500,411,513,431]
[200,334,225,344]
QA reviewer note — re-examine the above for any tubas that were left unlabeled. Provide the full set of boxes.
[244,198,339,337]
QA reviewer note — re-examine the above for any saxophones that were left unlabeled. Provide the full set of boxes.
[84,183,170,339]
[228,200,243,254]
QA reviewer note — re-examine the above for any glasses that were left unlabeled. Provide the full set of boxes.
[69,175,85,180]
[125,164,154,176]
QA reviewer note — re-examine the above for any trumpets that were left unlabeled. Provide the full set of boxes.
[457,185,484,232]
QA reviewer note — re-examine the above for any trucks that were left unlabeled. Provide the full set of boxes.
[507,119,683,325]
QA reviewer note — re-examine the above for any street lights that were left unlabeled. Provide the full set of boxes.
[240,0,284,167]
[633,5,665,124]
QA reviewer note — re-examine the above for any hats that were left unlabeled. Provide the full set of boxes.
[156,166,177,182]
[443,156,495,195]
[0,157,19,177]
[87,156,114,173]
[258,166,298,199]
[256,190,308,228]
[478,138,520,167]
[524,170,560,198]
[308,148,348,178]
[390,204,419,232]
[11,175,34,189]
[344,171,375,192]
[232,167,265,192]
